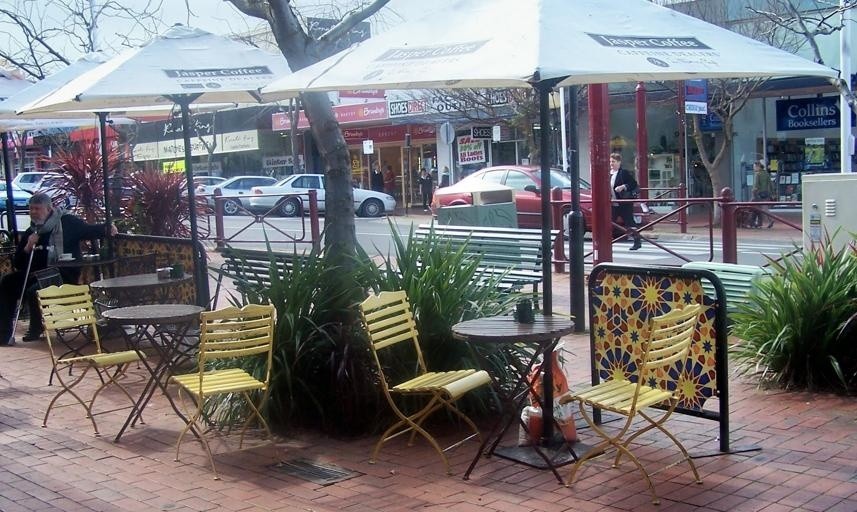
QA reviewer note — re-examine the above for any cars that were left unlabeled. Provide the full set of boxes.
[173,172,279,217]
[0,168,146,216]
[248,171,398,218]
[429,165,651,241]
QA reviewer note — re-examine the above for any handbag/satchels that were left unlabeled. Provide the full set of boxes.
[618,184,642,199]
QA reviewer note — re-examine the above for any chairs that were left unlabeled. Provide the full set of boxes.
[566,304,703,505]
[358,291,491,476]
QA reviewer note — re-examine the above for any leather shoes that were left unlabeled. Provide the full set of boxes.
[629,240,641,250]
[22,323,45,342]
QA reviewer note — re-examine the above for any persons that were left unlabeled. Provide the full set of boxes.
[381,165,395,196]
[415,168,432,212]
[606,153,642,250]
[371,164,383,193]
[0,193,119,344]
[752,161,775,228]
[437,166,450,189]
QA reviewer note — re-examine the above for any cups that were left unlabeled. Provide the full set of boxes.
[516,302,532,323]
[82,254,100,262]
[58,253,72,259]
[156,264,184,280]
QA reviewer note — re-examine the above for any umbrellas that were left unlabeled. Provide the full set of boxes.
[0,67,137,257]
[0,45,236,278]
[16,21,295,308]
[258,0,843,439]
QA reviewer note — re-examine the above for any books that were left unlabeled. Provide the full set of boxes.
[763,139,840,203]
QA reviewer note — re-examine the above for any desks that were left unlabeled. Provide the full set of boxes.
[449,312,578,484]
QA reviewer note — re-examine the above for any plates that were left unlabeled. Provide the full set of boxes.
[57,258,75,261]
